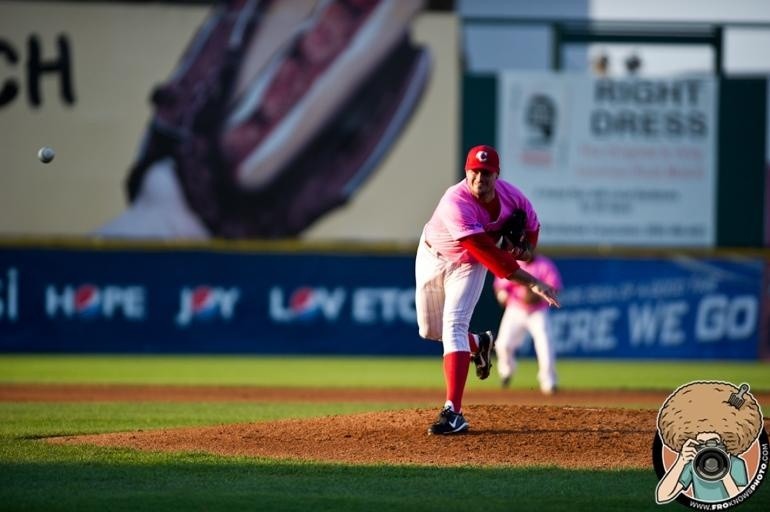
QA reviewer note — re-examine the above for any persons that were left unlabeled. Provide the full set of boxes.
[654,382,763,504]
[411,144,562,438]
[491,252,562,396]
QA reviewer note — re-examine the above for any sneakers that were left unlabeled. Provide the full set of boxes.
[474,330,494,380]
[428,405,470,435]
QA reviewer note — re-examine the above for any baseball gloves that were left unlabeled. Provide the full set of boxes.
[519,286,541,304]
[123,0,433,242]
[491,209,530,262]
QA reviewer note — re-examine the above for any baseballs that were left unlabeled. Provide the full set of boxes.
[37,146,54,164]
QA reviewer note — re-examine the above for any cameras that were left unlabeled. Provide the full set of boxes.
[692,440,732,483]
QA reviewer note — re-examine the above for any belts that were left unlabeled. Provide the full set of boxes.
[425,241,443,256]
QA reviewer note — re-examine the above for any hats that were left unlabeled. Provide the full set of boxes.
[465,144,500,174]
